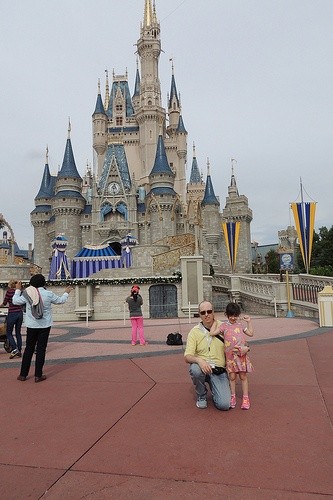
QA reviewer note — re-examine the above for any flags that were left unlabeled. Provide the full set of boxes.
[287,202,319,271]
[221,222,242,270]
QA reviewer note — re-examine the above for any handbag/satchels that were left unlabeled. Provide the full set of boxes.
[212,367,225,375]
[166,333,183,345]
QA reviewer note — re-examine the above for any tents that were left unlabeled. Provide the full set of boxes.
[72,244,123,281]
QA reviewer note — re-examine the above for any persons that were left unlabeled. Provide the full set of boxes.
[210,302,256,410]
[126,286,149,346]
[183,300,231,411]
[0,279,33,357]
[12,274,72,383]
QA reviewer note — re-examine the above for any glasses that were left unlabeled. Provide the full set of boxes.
[200,310,213,315]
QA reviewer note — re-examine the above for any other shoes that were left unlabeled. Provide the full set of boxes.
[132,344,136,345]
[35,375,47,382]
[17,376,26,381]
[140,342,149,345]
[9,349,21,359]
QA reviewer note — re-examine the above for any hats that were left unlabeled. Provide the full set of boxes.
[132,285,139,292]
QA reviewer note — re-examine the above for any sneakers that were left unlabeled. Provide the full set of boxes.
[196,397,207,408]
[241,397,250,409]
[231,394,236,408]
